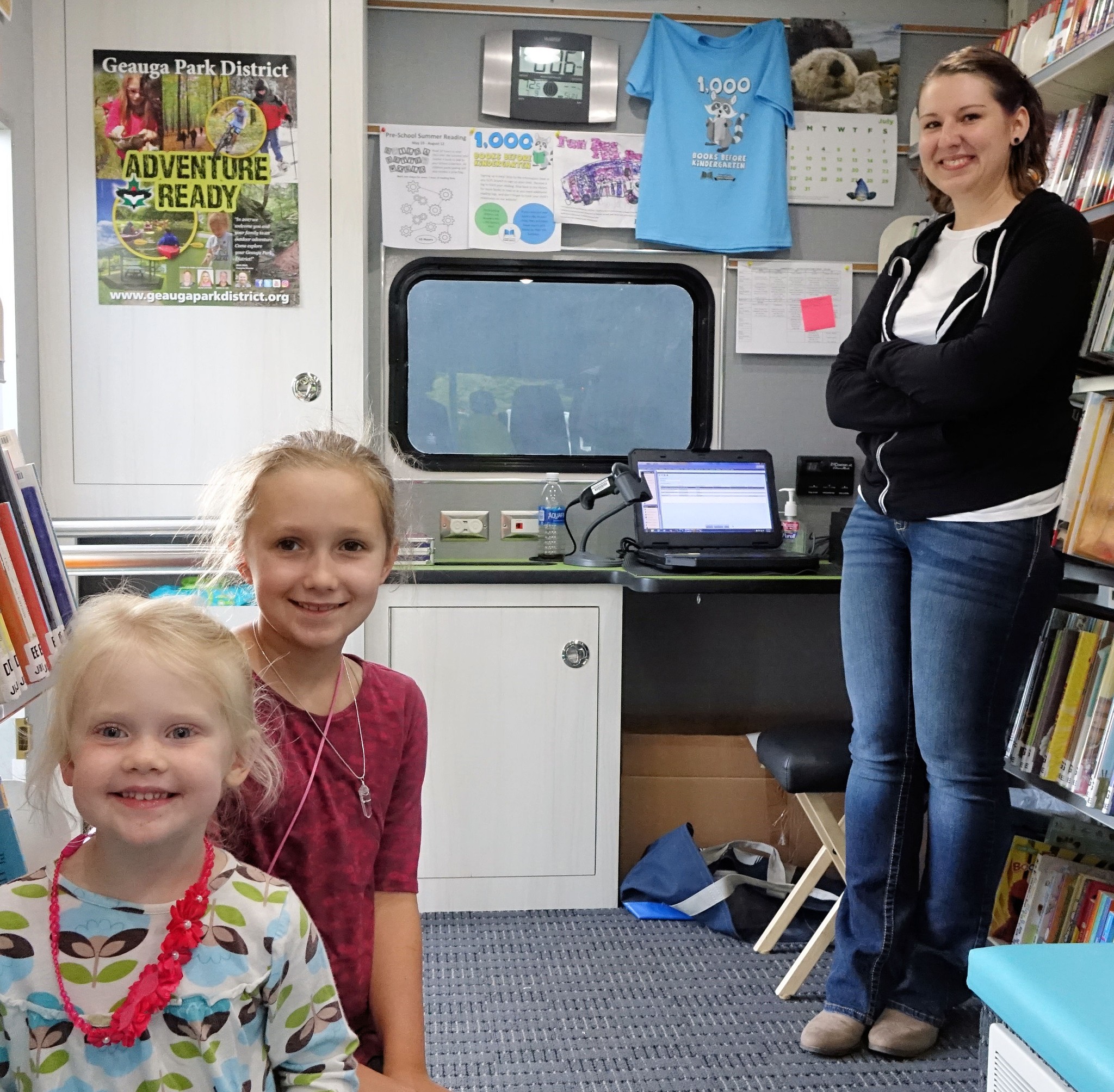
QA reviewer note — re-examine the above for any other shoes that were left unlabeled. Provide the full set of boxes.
[867,1003,941,1058]
[800,1008,867,1057]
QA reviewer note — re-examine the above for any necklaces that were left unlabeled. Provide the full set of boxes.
[49,835,214,1046]
[252,619,372,819]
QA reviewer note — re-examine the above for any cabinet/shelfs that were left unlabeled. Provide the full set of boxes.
[908,20,1114,947]
[360,574,622,911]
[31,1,370,533]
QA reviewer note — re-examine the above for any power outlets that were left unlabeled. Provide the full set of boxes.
[440,510,489,541]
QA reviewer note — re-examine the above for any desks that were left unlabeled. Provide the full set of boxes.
[621,564,843,595]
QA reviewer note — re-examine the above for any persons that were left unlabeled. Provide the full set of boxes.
[801,46,1095,1053]
[222,100,250,151]
[410,362,515,453]
[253,78,293,171]
[180,131,188,147]
[200,127,203,135]
[96,72,162,168]
[0,583,358,1092]
[207,431,450,1092]
[180,271,251,287]
[123,219,180,246]
[189,128,197,148]
[201,210,233,269]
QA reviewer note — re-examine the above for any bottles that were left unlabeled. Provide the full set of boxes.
[538,472,566,558]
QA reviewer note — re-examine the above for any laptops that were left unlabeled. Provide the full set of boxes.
[628,449,820,573]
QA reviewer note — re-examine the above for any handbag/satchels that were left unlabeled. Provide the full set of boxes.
[619,821,846,944]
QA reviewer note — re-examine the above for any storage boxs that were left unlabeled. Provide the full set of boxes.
[621,713,928,887]
[394,533,437,566]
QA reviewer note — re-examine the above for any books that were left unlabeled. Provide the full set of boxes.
[0,298,77,884]
[988,0,1114,944]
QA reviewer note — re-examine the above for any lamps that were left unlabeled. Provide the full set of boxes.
[563,463,654,567]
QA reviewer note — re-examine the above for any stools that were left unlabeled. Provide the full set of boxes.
[752,723,853,1002]
[967,944,1114,1092]
